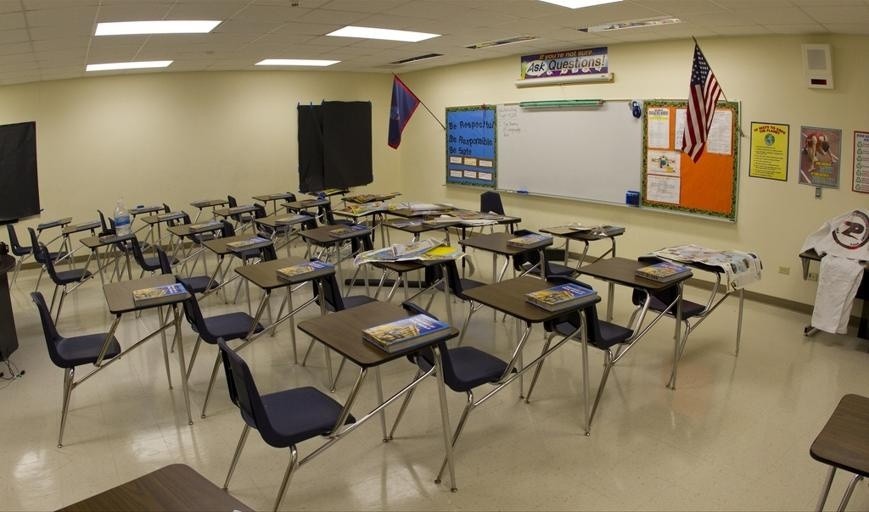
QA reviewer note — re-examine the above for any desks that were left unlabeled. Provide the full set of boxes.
[810,391,868,510]
[796,250,869,342]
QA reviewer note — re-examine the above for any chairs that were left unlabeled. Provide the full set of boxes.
[7,221,121,452]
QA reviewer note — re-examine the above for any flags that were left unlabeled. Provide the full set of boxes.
[679,44,723,164]
[388,73,421,151]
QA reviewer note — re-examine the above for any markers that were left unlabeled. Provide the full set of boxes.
[517,190,528,193]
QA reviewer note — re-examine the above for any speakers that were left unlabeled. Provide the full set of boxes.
[0,121,40,221]
[297,102,325,194]
[321,99,374,190]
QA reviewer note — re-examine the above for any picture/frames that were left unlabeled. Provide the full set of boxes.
[748,121,790,182]
[851,130,868,194]
[798,125,842,189]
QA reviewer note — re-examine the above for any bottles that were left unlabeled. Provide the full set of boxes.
[114,199,131,237]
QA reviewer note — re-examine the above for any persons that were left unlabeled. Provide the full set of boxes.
[801,131,838,173]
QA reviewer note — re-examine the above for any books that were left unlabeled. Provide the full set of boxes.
[525,280,601,312]
[360,314,452,354]
[36,186,624,267]
[277,257,336,284]
[132,282,190,308]
[635,260,694,284]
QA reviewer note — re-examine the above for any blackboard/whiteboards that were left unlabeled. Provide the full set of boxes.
[496,99,645,208]
